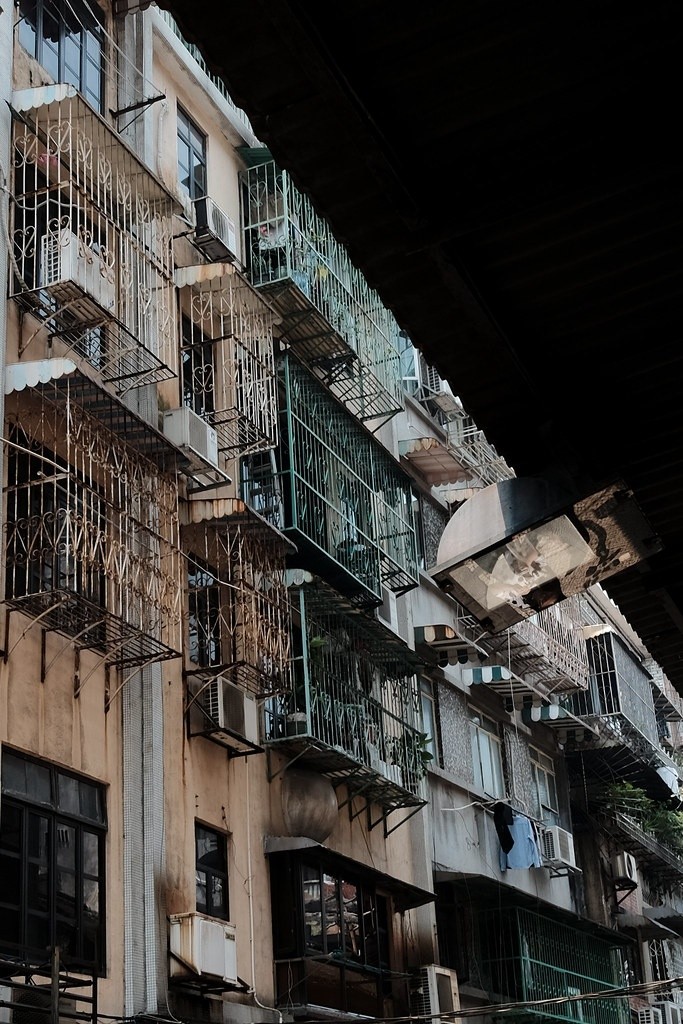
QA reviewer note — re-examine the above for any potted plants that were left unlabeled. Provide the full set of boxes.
[389,730,434,795]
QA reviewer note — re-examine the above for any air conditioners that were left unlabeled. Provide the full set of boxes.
[414,963,463,1024]
[638,1001,682,1024]
[201,675,259,753]
[612,851,638,887]
[40,228,115,324]
[540,826,576,871]
[163,406,218,474]
[426,364,463,412]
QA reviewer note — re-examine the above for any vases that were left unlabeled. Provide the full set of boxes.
[274,264,287,281]
